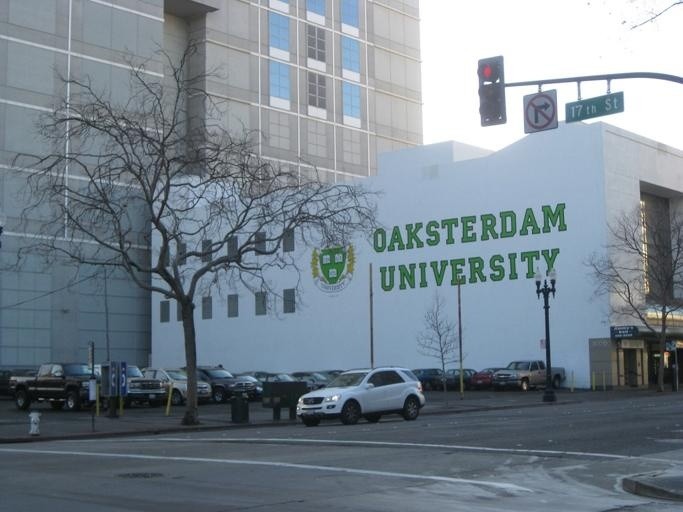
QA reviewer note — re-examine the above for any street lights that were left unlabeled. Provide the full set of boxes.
[534,266,559,402]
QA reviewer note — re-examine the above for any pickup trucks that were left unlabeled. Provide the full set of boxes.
[492,360,565,391]
[8,362,105,410]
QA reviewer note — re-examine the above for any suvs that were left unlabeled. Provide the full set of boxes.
[296,366,426,427]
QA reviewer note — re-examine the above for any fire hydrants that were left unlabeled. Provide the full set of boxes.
[28,412,42,435]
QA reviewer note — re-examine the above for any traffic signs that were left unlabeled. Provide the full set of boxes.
[565,91,625,124]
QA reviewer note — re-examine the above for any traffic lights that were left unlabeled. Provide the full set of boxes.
[477,56,506,127]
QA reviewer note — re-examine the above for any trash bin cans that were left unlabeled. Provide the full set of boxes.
[230,390,249,424]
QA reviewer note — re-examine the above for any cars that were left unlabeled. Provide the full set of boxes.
[412,368,506,389]
[93,364,344,407]
